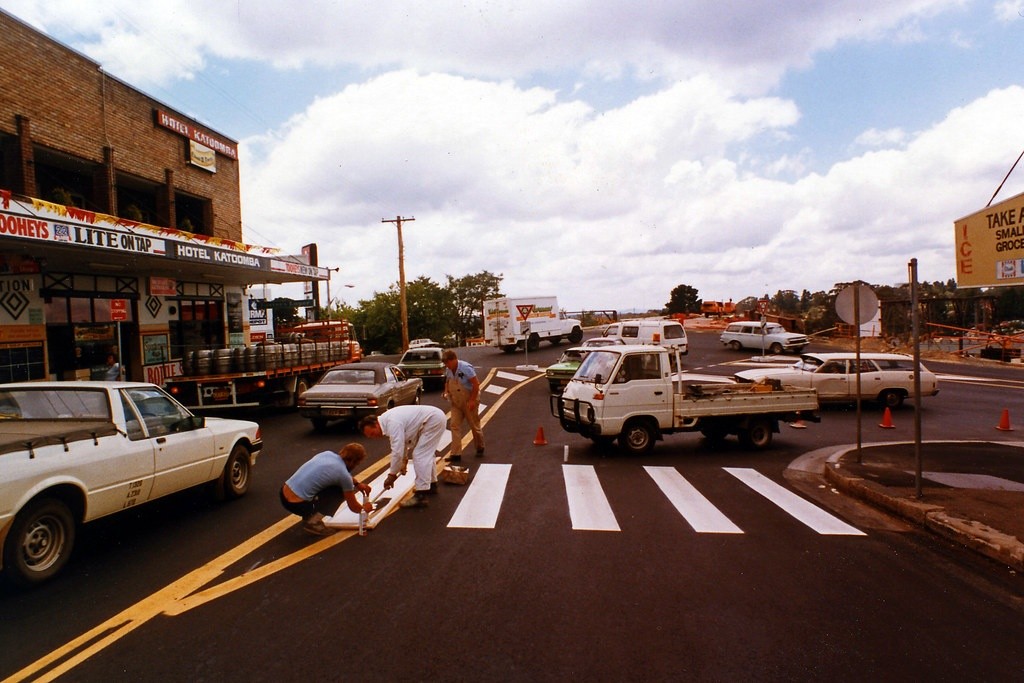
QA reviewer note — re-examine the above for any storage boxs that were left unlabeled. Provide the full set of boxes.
[64,369,90,381]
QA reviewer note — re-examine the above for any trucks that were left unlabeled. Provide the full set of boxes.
[551,345,821,462]
[165,320,363,415]
[483,296,584,354]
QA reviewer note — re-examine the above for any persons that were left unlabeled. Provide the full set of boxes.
[76,344,123,381]
[357,405,447,508]
[280,443,372,534]
[441,350,485,462]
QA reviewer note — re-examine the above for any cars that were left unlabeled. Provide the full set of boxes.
[545,347,598,392]
[581,337,626,347]
[409,340,439,348]
[719,321,810,354]
[395,348,447,383]
[298,362,424,430]
[734,351,940,412]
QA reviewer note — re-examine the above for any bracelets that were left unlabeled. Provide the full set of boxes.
[354,482,360,486]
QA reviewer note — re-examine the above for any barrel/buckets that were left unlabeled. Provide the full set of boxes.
[183,339,350,375]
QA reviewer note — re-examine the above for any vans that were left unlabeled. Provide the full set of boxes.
[601,319,688,357]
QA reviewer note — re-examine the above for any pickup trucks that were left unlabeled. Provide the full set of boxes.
[0,382,263,591]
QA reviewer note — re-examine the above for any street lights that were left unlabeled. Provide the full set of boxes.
[328,284,356,321]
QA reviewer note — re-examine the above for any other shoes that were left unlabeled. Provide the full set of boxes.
[400,493,429,507]
[475,447,484,457]
[303,521,335,536]
[444,454,461,463]
[424,479,440,494]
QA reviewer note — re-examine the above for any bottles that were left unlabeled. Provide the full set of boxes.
[359,508,368,536]
[563,443,569,462]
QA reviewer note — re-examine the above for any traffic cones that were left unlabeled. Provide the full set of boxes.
[995,408,1015,431]
[878,407,897,429]
[532,425,549,446]
[788,412,808,429]
